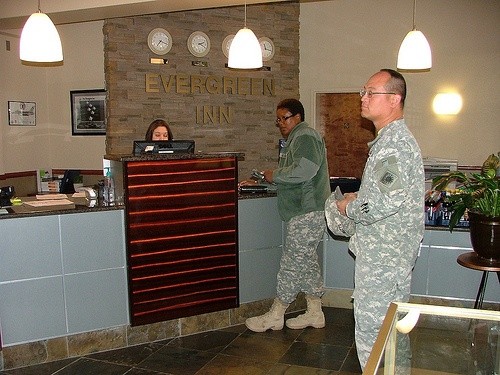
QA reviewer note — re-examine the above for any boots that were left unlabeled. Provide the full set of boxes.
[285,295,326,329]
[245,297,290,332]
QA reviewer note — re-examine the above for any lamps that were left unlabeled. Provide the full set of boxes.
[19,0,64,67]
[396,0,433,73]
[227,0,263,69]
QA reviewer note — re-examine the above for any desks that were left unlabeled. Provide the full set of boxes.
[456,253,500,346]
[361,301,500,375]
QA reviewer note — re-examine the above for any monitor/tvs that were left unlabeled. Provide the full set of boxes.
[132,139,195,157]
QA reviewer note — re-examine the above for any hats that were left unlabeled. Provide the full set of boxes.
[325,186,355,237]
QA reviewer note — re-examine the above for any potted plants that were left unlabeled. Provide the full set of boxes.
[429,152,499,263]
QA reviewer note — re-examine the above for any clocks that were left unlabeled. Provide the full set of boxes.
[259,37,275,61]
[147,27,173,56]
[187,31,211,58]
[221,34,236,59]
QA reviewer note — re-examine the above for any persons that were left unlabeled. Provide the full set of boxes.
[145,119,175,140]
[337,69,425,375]
[239,97,332,332]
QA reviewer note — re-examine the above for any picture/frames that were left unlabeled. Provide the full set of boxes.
[70,89,107,136]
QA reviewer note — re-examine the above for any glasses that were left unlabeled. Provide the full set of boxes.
[276,113,296,123]
[359,89,396,97]
